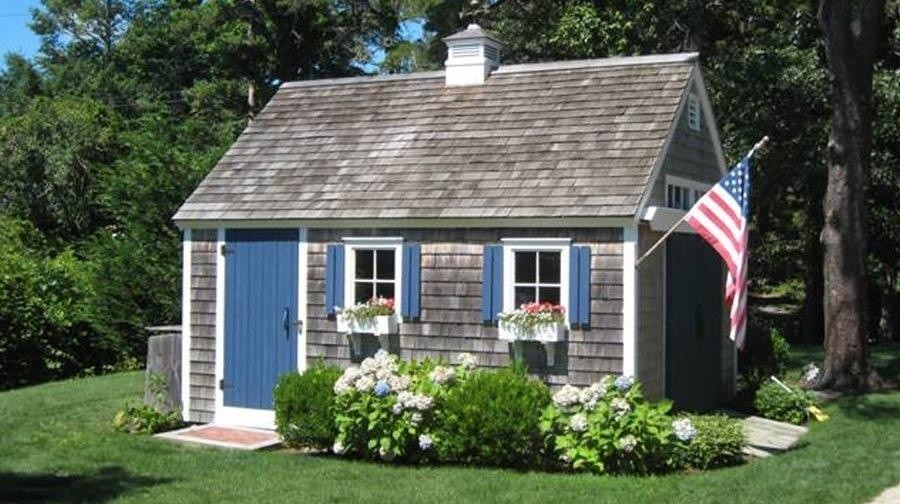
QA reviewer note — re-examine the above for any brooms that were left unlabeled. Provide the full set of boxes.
[770,375,829,423]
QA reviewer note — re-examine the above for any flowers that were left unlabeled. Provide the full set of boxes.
[336,295,395,337]
[496,302,567,344]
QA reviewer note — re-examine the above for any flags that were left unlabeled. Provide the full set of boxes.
[684,148,753,353]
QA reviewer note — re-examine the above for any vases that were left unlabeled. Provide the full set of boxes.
[337,316,397,334]
[498,323,564,342]
